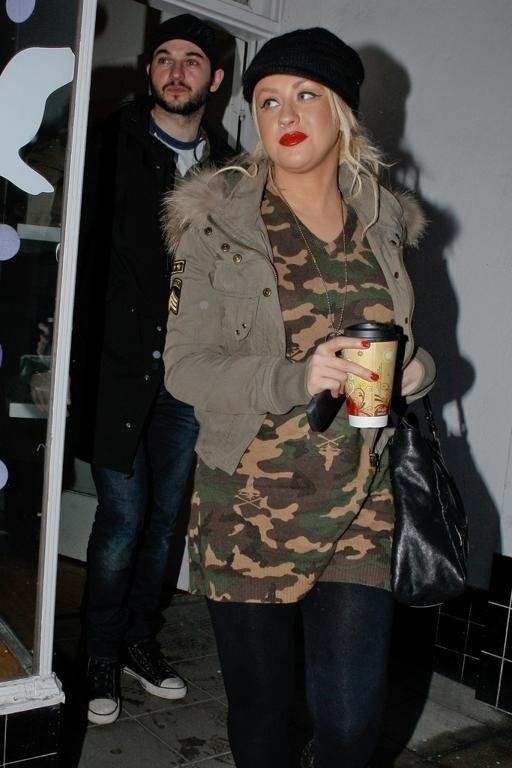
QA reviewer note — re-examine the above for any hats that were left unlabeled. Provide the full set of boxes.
[241,26,364,104]
[147,12,225,68]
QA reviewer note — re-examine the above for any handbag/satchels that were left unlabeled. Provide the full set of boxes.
[385,400,501,613]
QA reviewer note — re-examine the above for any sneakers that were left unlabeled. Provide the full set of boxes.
[84,667,122,728]
[122,656,186,702]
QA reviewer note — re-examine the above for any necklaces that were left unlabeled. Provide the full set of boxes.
[272,175,348,341]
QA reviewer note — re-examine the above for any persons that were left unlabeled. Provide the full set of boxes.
[74,15,235,726]
[161,25,439,768]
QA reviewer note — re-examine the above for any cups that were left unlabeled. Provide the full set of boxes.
[338,324,402,430]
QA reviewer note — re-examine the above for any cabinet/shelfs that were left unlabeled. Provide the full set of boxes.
[0,0,101,716]
